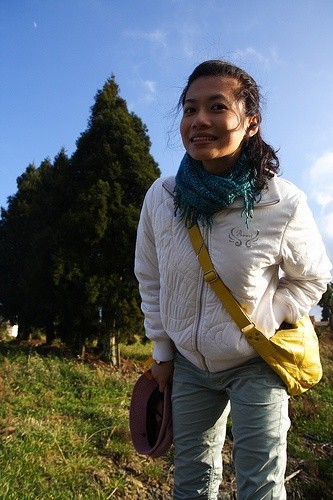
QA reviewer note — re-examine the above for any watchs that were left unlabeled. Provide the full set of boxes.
[153,360,165,365]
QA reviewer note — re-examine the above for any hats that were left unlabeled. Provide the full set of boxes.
[130,369,173,458]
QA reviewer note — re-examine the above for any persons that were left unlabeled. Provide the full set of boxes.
[129,60,333,500]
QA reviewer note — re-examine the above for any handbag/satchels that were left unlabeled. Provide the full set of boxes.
[266,313,322,395]
